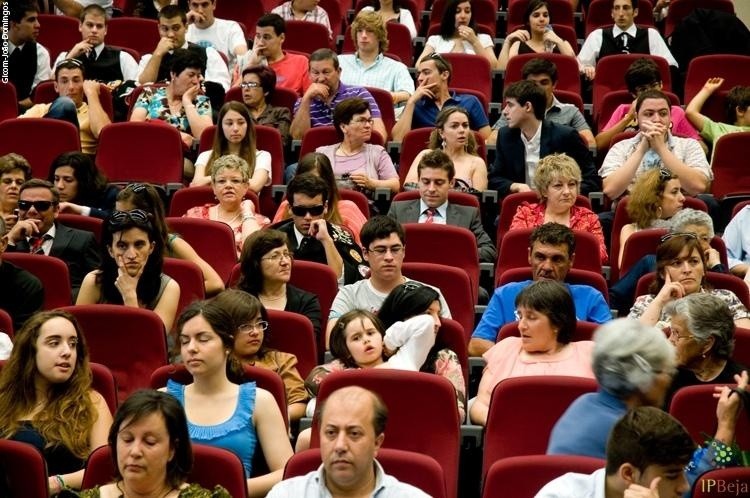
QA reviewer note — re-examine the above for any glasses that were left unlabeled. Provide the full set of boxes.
[658,169,671,193]
[237,203,422,334]
[56,59,83,67]
[429,52,446,65]
[660,231,699,244]
[17,182,155,228]
[240,82,261,89]
[351,118,375,126]
[672,329,694,344]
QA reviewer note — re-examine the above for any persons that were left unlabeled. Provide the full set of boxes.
[1,0,749,498]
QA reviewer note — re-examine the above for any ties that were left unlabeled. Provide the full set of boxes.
[621,32,631,55]
[88,47,97,61]
[426,208,436,223]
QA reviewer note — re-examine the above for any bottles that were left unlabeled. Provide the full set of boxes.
[544,24,554,53]
